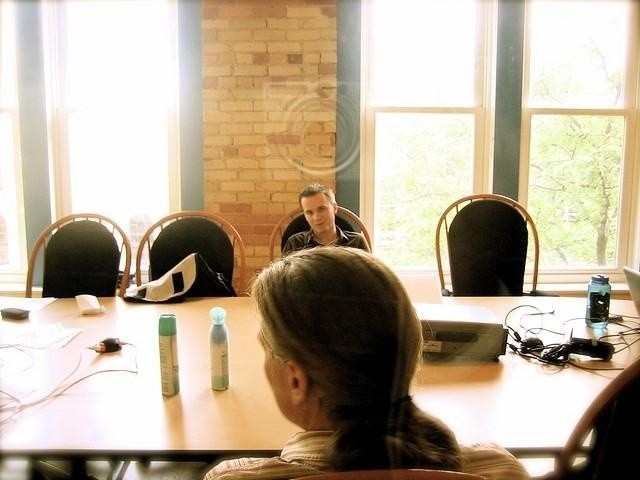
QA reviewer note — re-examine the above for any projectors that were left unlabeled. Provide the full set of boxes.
[411,301,508,362]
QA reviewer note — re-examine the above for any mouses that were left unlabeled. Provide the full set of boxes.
[521,338,543,353]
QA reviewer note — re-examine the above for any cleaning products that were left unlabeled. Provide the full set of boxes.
[159,313,179,395]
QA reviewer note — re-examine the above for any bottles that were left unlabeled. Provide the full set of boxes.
[585,273,612,330]
[158,312,181,398]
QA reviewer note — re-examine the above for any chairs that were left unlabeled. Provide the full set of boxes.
[25,213,132,298]
[434,193,541,296]
[552,359,639,480]
[135,210,246,296]
[269,205,374,259]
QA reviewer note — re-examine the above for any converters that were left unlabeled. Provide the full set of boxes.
[571,337,614,361]
[96,338,121,353]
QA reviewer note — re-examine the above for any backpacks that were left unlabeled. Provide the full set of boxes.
[123,253,238,303]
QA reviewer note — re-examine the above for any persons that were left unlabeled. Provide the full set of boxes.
[282,183,371,256]
[203,245,531,480]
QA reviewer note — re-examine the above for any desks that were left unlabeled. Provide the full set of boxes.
[1,294,640,461]
